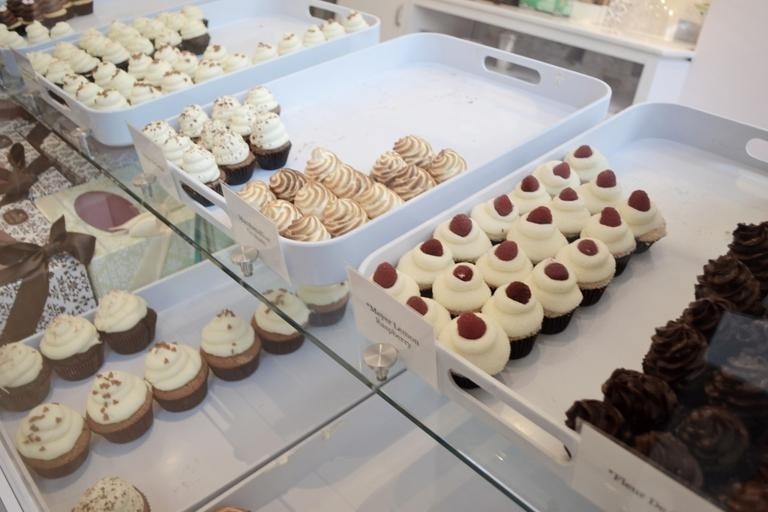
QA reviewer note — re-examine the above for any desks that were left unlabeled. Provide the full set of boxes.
[394,1,697,120]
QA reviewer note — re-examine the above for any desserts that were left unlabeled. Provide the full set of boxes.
[563,221,768,512]
[27,7,371,112]
[370,142,666,377]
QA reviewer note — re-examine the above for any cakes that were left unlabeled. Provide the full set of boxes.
[0,0,93,51]
[235,135,467,242]
[86,371,155,444]
[68,478,150,511]
[93,292,156,354]
[39,315,103,380]
[300,281,351,326]
[0,343,49,412]
[144,341,209,411]
[16,403,91,478]
[251,286,309,352]
[140,86,294,205]
[200,307,261,380]
